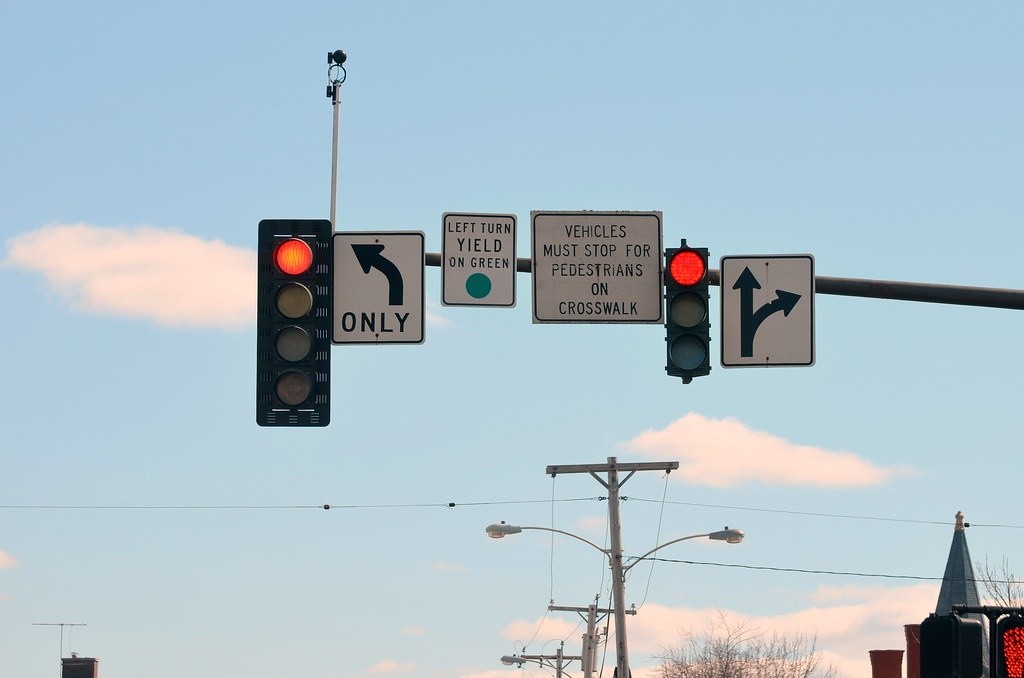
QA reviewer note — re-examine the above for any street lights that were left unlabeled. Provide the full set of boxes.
[485,524,744,678]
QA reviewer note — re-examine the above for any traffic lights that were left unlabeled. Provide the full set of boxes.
[920,614,983,677]
[664,248,710,376]
[257,218,334,428]
[996,616,1024,678]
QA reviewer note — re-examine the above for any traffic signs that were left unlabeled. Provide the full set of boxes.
[331,231,425,345]
